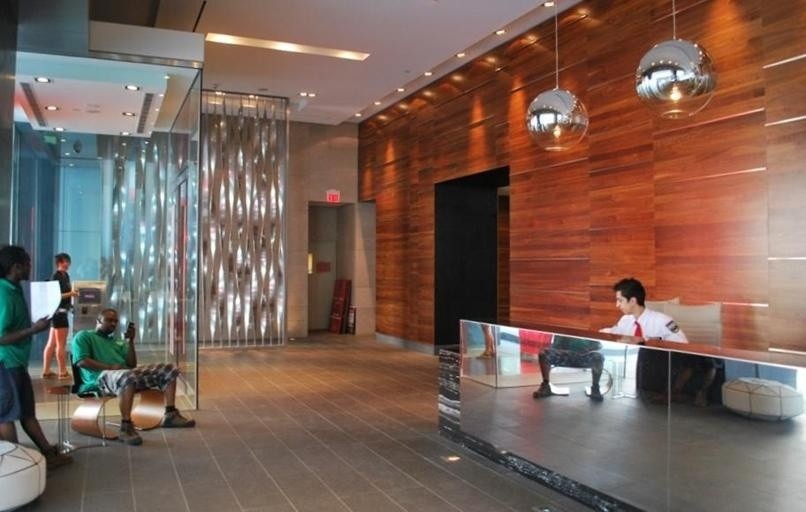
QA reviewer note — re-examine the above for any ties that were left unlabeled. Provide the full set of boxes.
[634,321,642,337]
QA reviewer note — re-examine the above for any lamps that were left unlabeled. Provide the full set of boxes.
[523,0,719,154]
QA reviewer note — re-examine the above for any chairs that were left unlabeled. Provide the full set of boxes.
[69,354,169,440]
[645,297,725,348]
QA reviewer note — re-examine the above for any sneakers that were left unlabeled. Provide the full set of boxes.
[160,410,196,428]
[590,386,604,402]
[117,422,143,445]
[41,371,71,380]
[653,387,688,403]
[45,446,74,470]
[475,351,496,359]
[533,382,551,398]
[694,390,706,408]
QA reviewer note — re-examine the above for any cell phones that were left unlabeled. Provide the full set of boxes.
[127,323,134,333]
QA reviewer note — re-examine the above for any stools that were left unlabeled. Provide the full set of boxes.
[1,439,49,511]
[721,378,806,423]
[548,363,615,397]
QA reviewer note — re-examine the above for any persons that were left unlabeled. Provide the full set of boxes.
[476,324,494,359]
[0,246,61,464]
[598,277,689,344]
[72,309,195,445]
[652,353,724,408]
[533,335,605,401]
[41,253,80,379]
